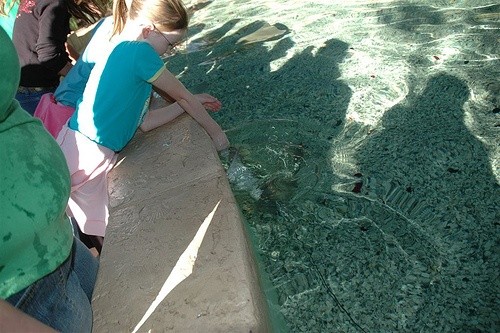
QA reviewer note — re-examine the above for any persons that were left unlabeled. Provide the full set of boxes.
[0,0,21,43]
[0,20,101,333]
[53,0,231,257]
[33,11,175,141]
[10,0,114,115]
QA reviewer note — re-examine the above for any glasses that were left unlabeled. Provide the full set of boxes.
[152,23,176,54]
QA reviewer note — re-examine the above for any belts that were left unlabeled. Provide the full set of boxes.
[17,85,47,93]
